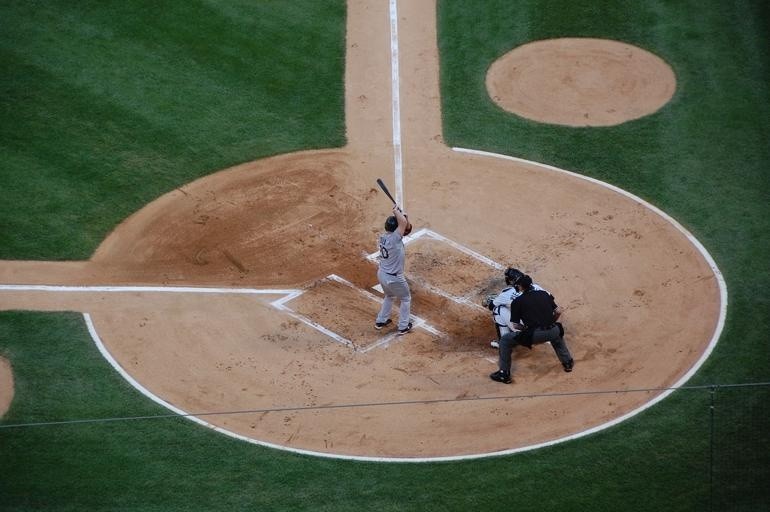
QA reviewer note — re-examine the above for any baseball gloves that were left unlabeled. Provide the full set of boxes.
[482,293,498,307]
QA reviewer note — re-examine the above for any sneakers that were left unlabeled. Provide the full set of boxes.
[489,368,513,384]
[397,322,412,334]
[374,318,392,329]
[491,339,500,348]
[563,358,574,372]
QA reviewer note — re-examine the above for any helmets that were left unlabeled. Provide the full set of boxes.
[384,216,398,232]
[505,268,532,292]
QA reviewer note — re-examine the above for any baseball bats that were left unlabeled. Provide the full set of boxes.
[377,179,401,212]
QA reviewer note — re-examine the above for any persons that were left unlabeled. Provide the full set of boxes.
[491,274,574,384]
[373,205,416,335]
[490,268,555,348]
